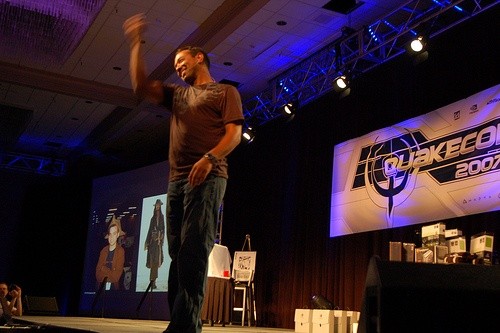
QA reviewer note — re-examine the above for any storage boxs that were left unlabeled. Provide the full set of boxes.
[295,309,361,333]
[390,223,494,265]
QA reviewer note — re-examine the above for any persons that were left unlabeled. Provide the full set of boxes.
[145,199,164,289]
[123,13,245,333]
[96,218,126,291]
[0,278,22,317]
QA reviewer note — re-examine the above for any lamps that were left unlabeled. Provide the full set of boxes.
[337,70,352,88]
[243,122,258,140]
[284,99,299,114]
[312,296,334,309]
[411,34,429,51]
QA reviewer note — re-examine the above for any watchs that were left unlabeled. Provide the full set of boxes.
[204,152,218,165]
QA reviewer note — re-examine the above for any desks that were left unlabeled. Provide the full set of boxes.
[202,277,232,327]
[378,261,500,333]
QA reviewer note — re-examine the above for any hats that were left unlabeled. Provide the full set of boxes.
[104,214,126,239]
[153,198,163,206]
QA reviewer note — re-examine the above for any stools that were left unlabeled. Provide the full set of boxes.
[232,269,253,327]
